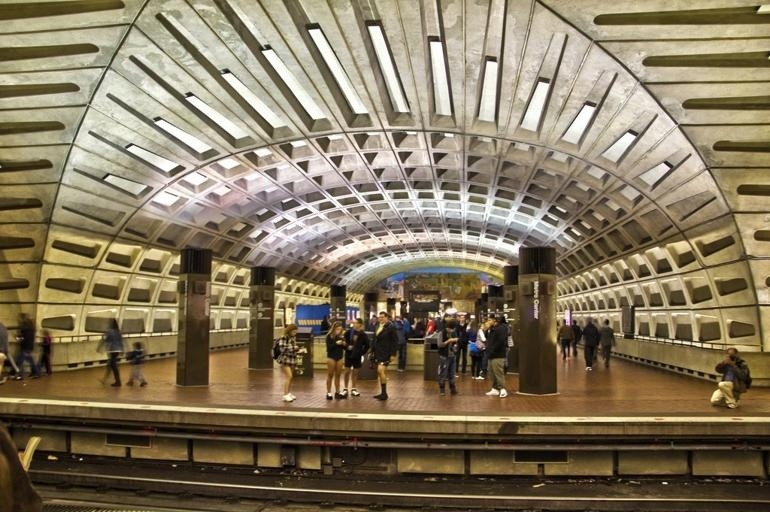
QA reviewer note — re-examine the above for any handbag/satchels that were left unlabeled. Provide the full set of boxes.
[370,355,377,369]
[476,340,486,350]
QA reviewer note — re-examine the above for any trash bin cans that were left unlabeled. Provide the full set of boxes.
[423,337,441,381]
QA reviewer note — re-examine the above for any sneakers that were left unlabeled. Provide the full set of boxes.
[471,375,508,398]
[282,388,387,402]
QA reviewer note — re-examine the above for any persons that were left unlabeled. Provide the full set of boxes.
[710,346,750,413]
[123,342,148,387]
[35,330,56,373]
[272,311,515,402]
[557,317,615,370]
[0,323,10,385]
[94,317,123,388]
[13,312,37,381]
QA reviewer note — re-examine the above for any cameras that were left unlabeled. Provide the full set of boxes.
[729,355,736,361]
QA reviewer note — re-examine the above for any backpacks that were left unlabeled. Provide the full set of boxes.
[273,335,287,359]
